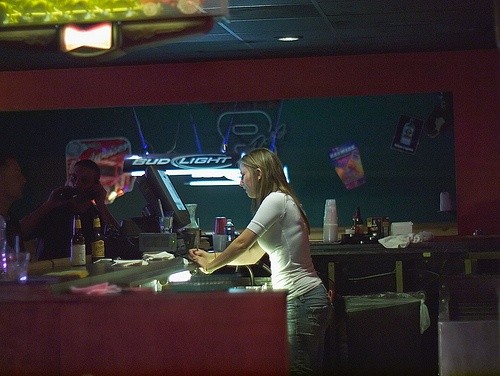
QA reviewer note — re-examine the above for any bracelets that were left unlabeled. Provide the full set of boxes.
[206,263,213,273]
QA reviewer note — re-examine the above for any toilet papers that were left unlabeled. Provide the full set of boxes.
[439,191,452,211]
[391,221,413,235]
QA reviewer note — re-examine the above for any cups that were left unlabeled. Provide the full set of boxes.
[214,216,227,235]
[7,253,29,280]
[323,199,339,243]
[158,217,174,234]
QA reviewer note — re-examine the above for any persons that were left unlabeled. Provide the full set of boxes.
[0,151,122,261]
[188,149,332,376]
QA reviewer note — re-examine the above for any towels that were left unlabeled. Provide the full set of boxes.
[378,231,415,248]
[411,230,434,243]
[142,250,175,260]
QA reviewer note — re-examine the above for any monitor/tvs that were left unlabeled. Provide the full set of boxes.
[145,166,192,229]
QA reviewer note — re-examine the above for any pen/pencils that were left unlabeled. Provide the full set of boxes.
[195,237,199,249]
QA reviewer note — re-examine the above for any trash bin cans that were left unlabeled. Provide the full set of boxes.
[330,291,426,376]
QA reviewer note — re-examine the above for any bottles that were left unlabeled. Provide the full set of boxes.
[225,219,235,246]
[91,213,105,263]
[353,206,363,226]
[0,214,9,275]
[382,216,390,238]
[70,212,87,267]
